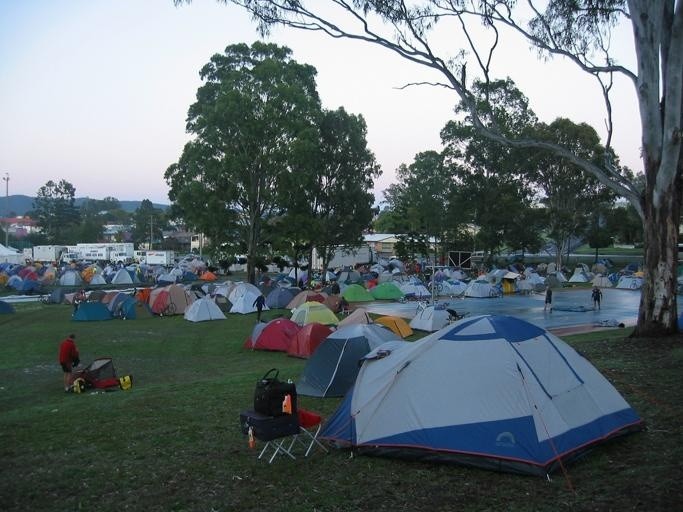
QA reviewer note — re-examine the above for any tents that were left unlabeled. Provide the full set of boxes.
[296,324,404,398]
[287,322,332,360]
[317,314,648,478]
[243,318,300,352]
[1,257,644,338]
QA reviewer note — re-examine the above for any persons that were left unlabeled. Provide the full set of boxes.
[60,335,80,393]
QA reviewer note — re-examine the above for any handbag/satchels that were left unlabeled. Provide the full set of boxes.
[239,410,301,441]
[254,368,297,417]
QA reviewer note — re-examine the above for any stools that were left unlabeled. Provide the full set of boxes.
[258,409,329,464]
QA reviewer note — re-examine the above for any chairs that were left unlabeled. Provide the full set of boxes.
[446,307,471,322]
